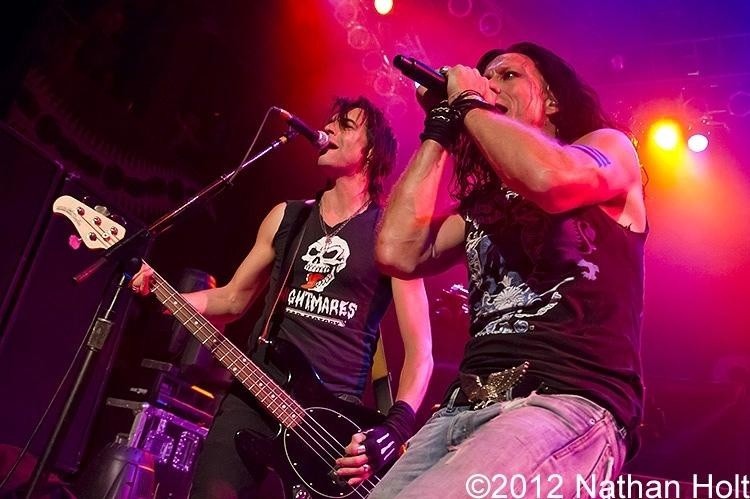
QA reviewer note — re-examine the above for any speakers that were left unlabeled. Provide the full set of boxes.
[61,267,266,498]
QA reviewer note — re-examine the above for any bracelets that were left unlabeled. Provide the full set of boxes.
[421,88,495,148]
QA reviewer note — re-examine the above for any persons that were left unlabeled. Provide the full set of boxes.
[366,42,650,499]
[127,96,435,499]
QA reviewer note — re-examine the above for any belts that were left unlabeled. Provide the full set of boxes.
[329,390,366,407]
[434,361,563,406]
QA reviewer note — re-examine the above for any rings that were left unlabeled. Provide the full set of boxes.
[358,445,365,454]
[132,284,139,291]
[363,465,368,472]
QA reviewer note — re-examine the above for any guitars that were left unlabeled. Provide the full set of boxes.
[52,193,405,498]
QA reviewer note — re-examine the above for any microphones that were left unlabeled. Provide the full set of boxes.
[279,109,329,149]
[393,55,448,100]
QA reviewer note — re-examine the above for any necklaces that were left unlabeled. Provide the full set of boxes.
[318,196,371,244]
